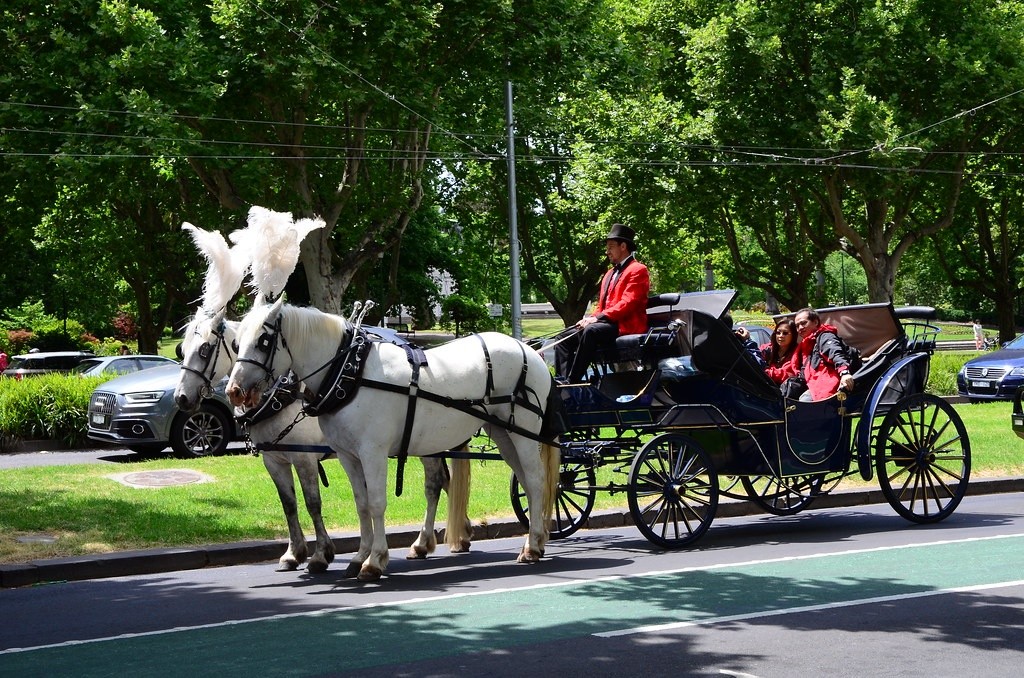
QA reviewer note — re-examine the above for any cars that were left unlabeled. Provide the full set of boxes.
[64,353,180,382]
[655,322,776,381]
[1,349,98,382]
[955,331,1024,405]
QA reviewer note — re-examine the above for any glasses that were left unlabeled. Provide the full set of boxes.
[776,330,792,335]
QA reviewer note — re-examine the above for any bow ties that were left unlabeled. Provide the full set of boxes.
[612,256,633,272]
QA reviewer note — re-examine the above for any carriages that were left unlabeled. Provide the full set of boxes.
[173,204,973,587]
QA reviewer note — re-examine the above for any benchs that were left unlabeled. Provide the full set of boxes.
[607,292,696,360]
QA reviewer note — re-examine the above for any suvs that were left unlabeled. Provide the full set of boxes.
[85,323,427,461]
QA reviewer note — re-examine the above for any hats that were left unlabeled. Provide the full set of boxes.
[600,224,637,251]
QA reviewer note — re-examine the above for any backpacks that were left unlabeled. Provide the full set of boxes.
[817,330,862,375]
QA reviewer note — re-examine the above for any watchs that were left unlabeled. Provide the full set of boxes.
[840,370,850,377]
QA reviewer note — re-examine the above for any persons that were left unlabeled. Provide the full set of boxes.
[686,309,863,403]
[0,348,9,374]
[120,345,130,355]
[973,319,984,350]
[552,223,648,386]
[983,331,999,350]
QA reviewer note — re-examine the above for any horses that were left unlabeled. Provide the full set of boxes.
[174,293,562,581]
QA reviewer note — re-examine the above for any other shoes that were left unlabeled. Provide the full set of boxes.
[554,376,568,385]
